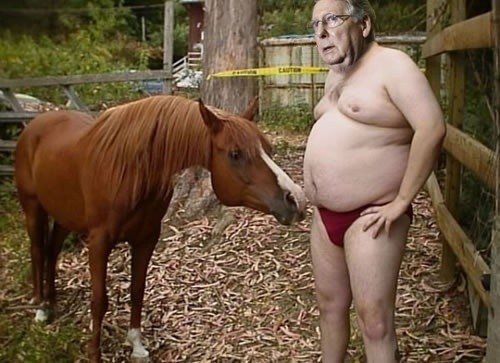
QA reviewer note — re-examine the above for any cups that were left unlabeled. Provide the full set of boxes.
[307,14,351,29]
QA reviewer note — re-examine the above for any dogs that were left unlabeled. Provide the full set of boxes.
[12,95,306,362]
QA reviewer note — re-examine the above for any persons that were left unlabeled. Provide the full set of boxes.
[303,0,447,363]
